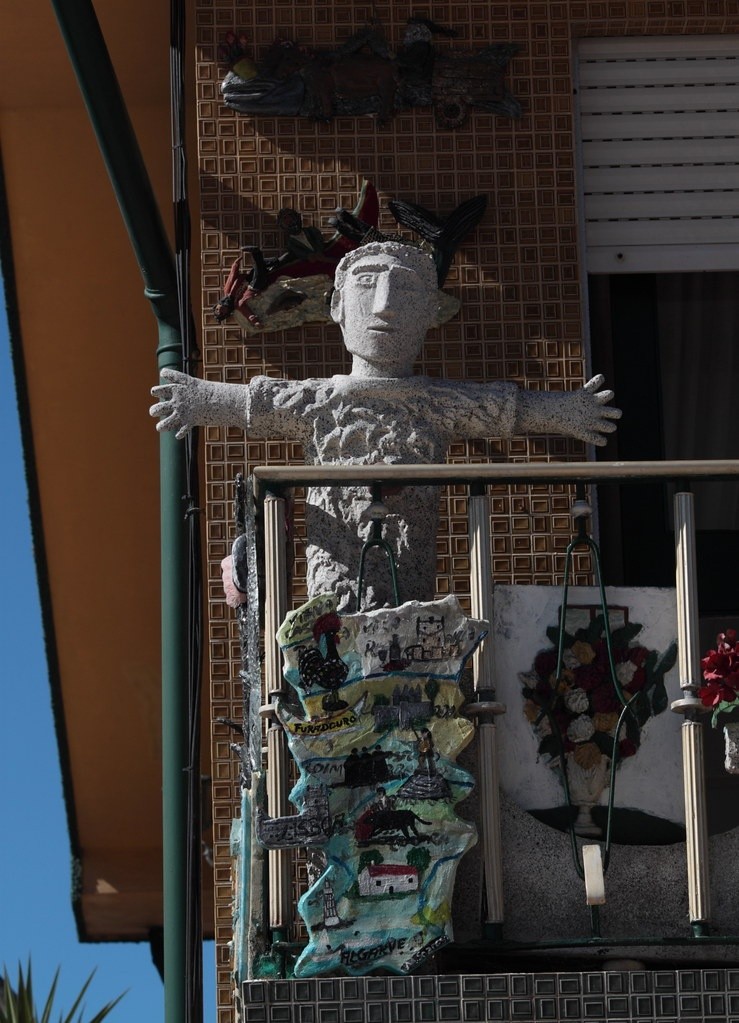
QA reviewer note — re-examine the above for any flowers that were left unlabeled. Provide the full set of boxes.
[697,626,739,728]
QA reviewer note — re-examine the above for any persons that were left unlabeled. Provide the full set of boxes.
[145,238,626,606]
